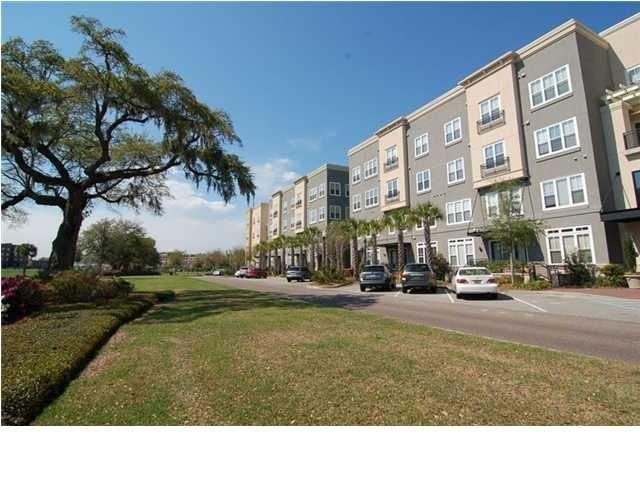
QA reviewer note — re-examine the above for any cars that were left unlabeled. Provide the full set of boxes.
[402,263,437,293]
[286,266,313,282]
[360,264,396,291]
[451,262,498,300]
[212,269,225,276]
[235,265,267,278]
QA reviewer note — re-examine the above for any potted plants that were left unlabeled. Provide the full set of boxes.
[622,232,640,289]
